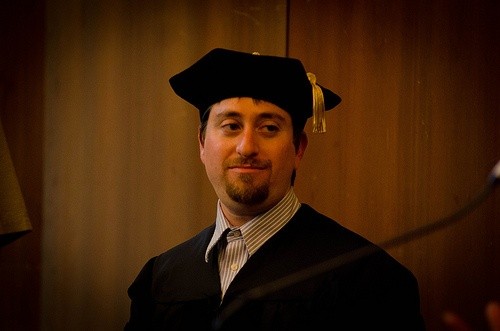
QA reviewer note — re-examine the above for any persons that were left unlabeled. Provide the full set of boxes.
[125,47,422,331]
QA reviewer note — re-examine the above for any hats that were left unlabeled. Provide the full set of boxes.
[165,46,343,142]
